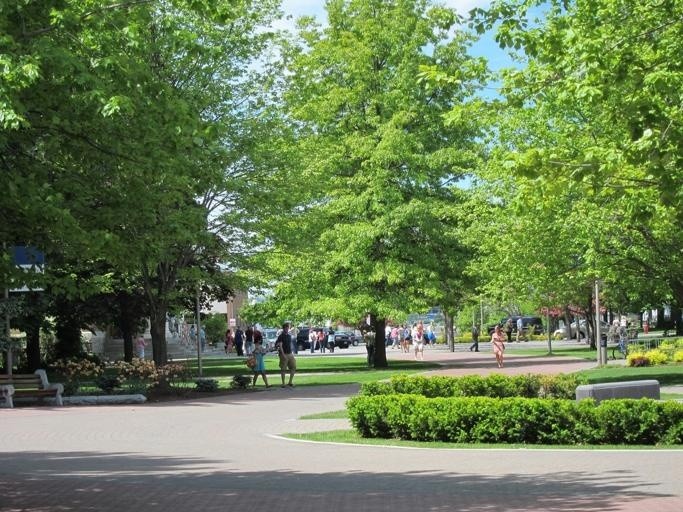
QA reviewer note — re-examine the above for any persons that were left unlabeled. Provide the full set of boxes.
[276,324,301,354]
[469,323,483,352]
[181,320,206,352]
[252,343,271,388]
[226,326,263,356]
[277,323,297,388]
[136,335,148,362]
[386,319,436,361]
[308,326,335,353]
[362,325,376,366]
[491,325,505,368]
[505,317,523,343]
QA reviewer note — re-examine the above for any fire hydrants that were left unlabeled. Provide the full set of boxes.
[643,320,649,334]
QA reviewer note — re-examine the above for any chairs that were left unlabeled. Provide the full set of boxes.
[0,367,64,407]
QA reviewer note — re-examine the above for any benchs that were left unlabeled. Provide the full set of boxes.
[606,338,626,361]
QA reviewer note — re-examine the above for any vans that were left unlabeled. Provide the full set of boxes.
[488,316,543,336]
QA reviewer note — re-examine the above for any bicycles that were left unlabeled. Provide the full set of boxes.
[612,338,628,360]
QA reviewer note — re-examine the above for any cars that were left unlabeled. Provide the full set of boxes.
[553,319,611,339]
[261,327,364,353]
